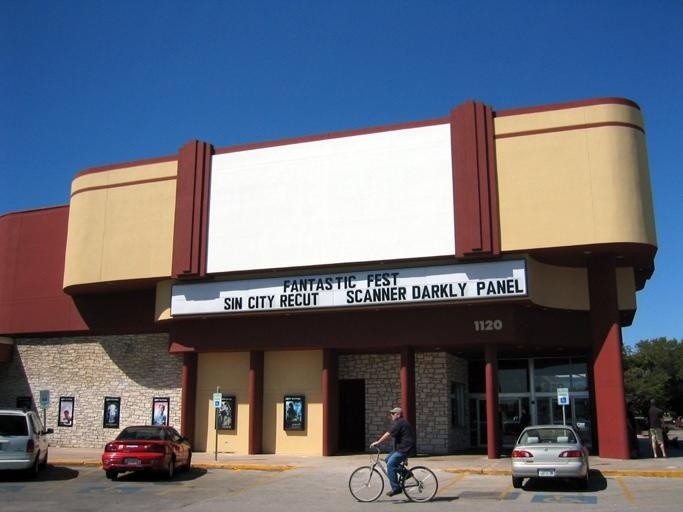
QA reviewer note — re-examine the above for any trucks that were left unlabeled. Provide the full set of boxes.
[662,412,672,424]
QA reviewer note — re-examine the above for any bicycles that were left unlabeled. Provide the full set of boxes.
[346,441,439,504]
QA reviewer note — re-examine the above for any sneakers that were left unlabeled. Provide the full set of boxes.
[400,473,412,480]
[387,491,402,496]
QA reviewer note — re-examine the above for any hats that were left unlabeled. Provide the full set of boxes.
[391,407,402,413]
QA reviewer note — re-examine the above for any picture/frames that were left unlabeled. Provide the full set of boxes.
[102,395,121,430]
[281,394,305,432]
[214,395,236,431]
[56,396,74,428]
[150,396,170,429]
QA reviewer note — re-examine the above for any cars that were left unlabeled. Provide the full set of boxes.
[99,423,192,483]
[507,424,590,486]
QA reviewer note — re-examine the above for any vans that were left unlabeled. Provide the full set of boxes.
[0,405,54,479]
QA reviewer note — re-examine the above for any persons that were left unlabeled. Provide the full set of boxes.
[153,404,165,425]
[286,403,295,417]
[643,397,668,458]
[61,410,70,424]
[624,401,641,459]
[370,407,414,498]
[106,402,117,424]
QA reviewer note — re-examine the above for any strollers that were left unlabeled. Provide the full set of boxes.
[646,430,669,458]
